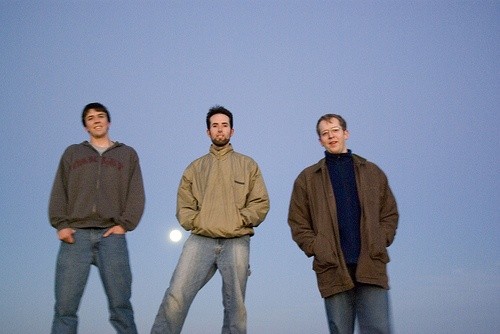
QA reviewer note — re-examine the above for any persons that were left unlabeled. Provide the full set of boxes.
[287,112,400,334]
[48,102,146,334]
[149,105,270,334]
